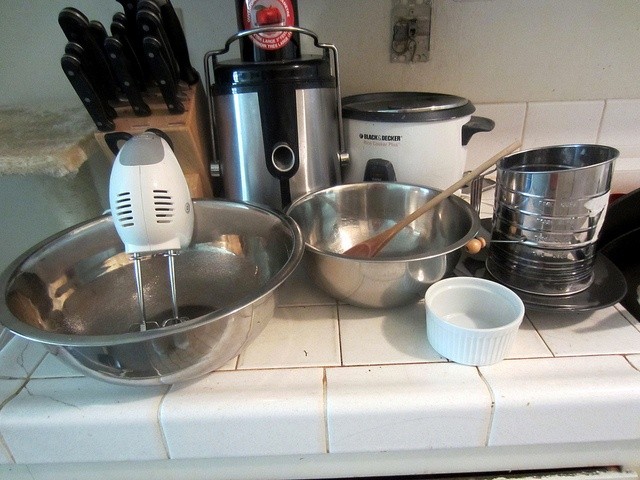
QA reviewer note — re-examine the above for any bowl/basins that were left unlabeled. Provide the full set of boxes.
[1,198,305,387]
[289,182,480,310]
[423,277,525,368]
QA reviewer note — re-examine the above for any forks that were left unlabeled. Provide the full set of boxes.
[472,177,483,215]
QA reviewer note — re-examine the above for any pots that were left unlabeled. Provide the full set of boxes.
[341,91,496,197]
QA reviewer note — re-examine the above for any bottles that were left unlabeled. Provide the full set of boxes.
[237,0,301,61]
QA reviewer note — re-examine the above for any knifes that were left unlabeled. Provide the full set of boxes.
[59,1,199,131]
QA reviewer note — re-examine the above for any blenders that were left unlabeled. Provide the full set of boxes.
[109,134,195,333]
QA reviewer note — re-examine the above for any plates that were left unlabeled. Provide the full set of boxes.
[453,217,629,311]
